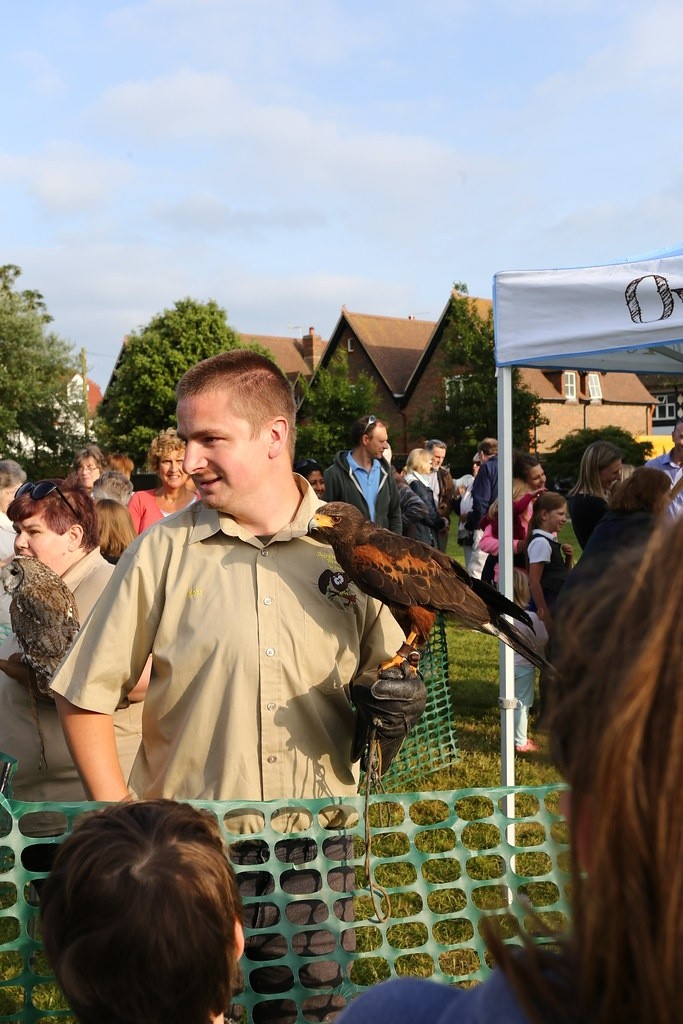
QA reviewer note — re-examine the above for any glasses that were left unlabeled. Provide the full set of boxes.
[472,460,480,466]
[293,458,317,471]
[14,482,79,520]
[363,415,376,434]
[76,467,97,474]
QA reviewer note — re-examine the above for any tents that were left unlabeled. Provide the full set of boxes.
[492,249,683,914]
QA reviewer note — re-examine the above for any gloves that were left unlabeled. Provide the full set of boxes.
[349,664,428,774]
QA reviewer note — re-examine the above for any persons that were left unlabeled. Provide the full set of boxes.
[0,348,683,1024]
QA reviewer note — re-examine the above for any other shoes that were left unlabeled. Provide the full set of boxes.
[514,738,543,752]
